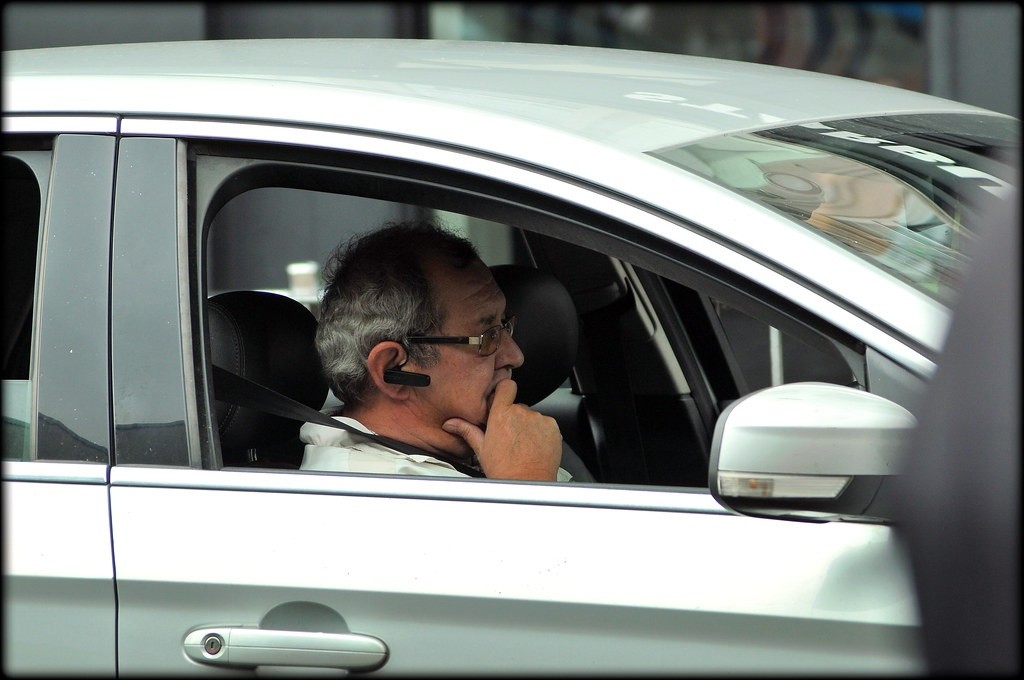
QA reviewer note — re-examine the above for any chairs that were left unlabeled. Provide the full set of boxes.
[485,262,594,484]
[204,291,330,474]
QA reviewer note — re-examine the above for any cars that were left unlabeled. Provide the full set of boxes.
[0,31,1023,679]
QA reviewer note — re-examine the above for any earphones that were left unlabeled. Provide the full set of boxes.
[383,364,430,387]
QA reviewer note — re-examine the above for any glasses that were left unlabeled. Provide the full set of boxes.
[407,315,516,357]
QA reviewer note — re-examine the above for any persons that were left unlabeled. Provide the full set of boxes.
[300,222,577,482]
